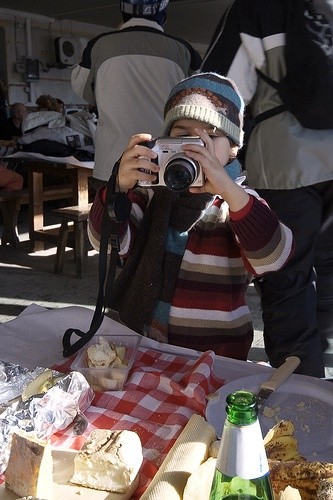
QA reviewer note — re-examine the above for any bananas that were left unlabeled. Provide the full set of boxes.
[266,459,333,500]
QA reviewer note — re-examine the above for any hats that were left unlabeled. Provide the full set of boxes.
[163,72,245,148]
[120,0,169,27]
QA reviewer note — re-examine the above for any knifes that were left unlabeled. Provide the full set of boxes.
[256,356,300,407]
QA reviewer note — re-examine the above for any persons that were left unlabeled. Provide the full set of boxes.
[70,0,203,194]
[226,0,333,382]
[88,73,295,362]
[0,95,65,191]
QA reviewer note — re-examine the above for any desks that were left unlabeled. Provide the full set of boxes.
[0,151,98,262]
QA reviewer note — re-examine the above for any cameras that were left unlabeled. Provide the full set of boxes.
[137,136,205,192]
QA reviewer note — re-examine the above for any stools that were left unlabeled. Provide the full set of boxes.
[51,203,125,279]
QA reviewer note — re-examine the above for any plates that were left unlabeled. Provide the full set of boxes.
[0,445,139,500]
[206,372,333,462]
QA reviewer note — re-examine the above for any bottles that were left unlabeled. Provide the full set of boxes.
[209,391,274,500]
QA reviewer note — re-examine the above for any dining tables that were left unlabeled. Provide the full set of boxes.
[0,303,333,500]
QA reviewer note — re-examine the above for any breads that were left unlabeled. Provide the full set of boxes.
[87,336,128,390]
[5,431,55,500]
[139,414,221,500]
[21,371,52,401]
[70,429,143,494]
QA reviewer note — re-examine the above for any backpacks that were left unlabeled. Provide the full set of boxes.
[247,0,333,129]
